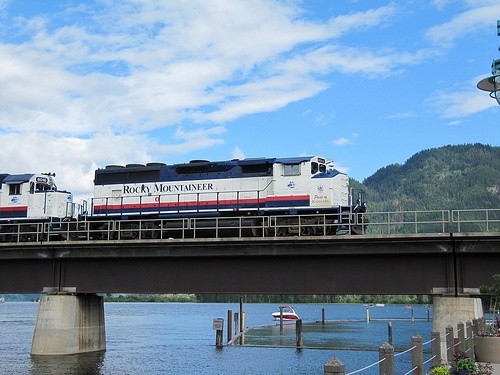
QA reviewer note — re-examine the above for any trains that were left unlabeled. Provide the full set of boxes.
[93,155,369,240]
[0,171,76,243]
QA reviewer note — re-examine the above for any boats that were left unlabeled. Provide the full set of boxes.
[375,304,385,307]
[272,305,301,321]
